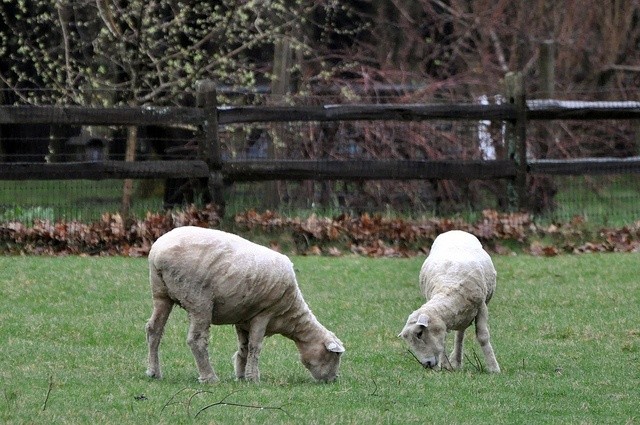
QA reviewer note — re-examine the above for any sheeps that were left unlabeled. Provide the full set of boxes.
[397,230,502,373]
[145,226,346,388]
